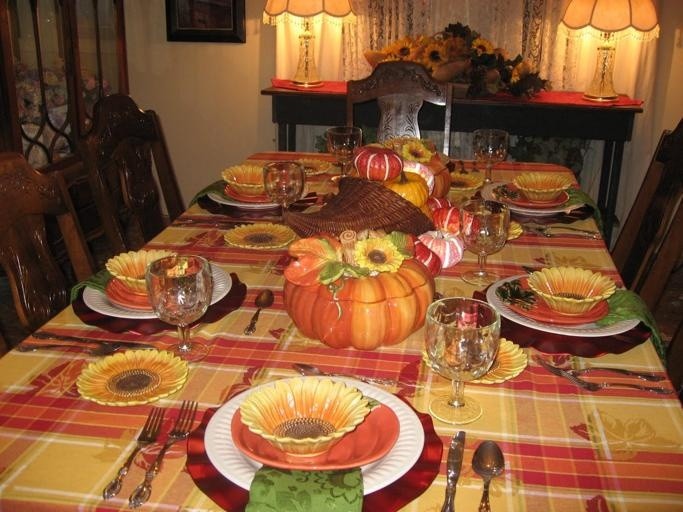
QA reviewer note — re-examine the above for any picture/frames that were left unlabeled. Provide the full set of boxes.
[166,0,247,46]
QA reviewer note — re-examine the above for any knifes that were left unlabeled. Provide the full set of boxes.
[442,431,464,511]
[26,331,159,352]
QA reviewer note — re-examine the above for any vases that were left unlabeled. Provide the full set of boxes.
[441,78,499,100]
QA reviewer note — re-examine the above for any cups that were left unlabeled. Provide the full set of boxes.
[262,160,306,223]
[456,199,512,290]
[325,124,363,185]
[144,255,216,362]
[420,296,504,427]
[471,128,510,193]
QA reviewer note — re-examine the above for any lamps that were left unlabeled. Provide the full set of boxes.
[553,0,663,104]
[263,1,361,87]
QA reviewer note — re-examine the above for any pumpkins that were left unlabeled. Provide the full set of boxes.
[433,206,473,240]
[351,147,404,181]
[409,236,441,278]
[418,230,464,269]
[282,229,436,352]
[383,171,429,208]
[347,140,451,199]
[426,197,452,212]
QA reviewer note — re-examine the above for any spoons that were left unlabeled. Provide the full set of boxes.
[470,441,507,512]
[12,341,122,357]
[242,288,274,336]
[527,224,601,235]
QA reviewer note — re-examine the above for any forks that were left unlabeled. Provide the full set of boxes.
[532,357,667,383]
[557,369,676,397]
[101,406,167,501]
[529,228,603,243]
[126,398,200,508]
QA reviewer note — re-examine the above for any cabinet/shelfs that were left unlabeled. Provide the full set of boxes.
[1,1,130,265]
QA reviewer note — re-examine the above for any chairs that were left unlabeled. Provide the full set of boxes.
[348,59,450,154]
[83,92,187,253]
[610,118,683,312]
[663,318,683,406]
[0,150,94,352]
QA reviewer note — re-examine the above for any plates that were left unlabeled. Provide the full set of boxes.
[297,157,330,177]
[449,172,482,193]
[480,181,587,217]
[496,182,570,210]
[82,263,233,321]
[497,276,610,325]
[205,184,281,211]
[422,340,529,383]
[75,342,191,411]
[485,274,643,341]
[106,276,152,313]
[506,219,522,244]
[221,222,297,251]
[201,373,428,499]
[223,183,274,205]
[228,397,400,475]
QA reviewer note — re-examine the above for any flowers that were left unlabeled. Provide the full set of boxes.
[363,23,555,100]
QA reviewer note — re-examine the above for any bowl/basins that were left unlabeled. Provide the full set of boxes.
[240,375,371,456]
[512,170,572,202]
[527,266,618,316]
[219,164,269,197]
[104,247,179,297]
